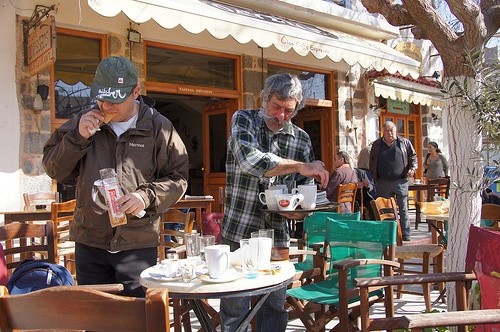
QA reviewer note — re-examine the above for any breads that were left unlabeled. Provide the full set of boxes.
[106,198,128,228]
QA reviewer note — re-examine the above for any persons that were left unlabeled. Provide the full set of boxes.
[322,150,359,202]
[41,55,189,299]
[369,121,418,241]
[218,71,330,332]
[422,142,450,202]
[348,153,377,222]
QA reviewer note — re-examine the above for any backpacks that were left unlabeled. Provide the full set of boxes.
[6,260,75,295]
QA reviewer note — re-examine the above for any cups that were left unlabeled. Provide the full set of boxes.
[270,246,290,261]
[240,238,258,279]
[258,228,275,249]
[414,179,421,184]
[203,245,230,279]
[161,258,179,278]
[184,233,200,262]
[254,237,272,274]
[275,193,304,211]
[259,189,283,211]
[292,184,317,210]
[420,176,426,185]
[279,180,296,194]
[200,235,215,263]
[251,232,268,239]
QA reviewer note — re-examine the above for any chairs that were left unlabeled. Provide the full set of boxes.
[0,175,500,332]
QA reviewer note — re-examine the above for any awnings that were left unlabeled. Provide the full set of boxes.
[85,0,419,81]
[365,70,446,108]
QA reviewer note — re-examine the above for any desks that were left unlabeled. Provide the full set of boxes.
[0,207,75,274]
[408,182,427,210]
[140,252,295,332]
[169,196,215,236]
[420,210,449,273]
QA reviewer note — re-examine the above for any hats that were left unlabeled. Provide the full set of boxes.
[90,55,138,104]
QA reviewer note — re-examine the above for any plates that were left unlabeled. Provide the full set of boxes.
[202,271,240,283]
[148,272,183,281]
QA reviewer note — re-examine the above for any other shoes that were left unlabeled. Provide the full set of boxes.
[403,235,410,241]
